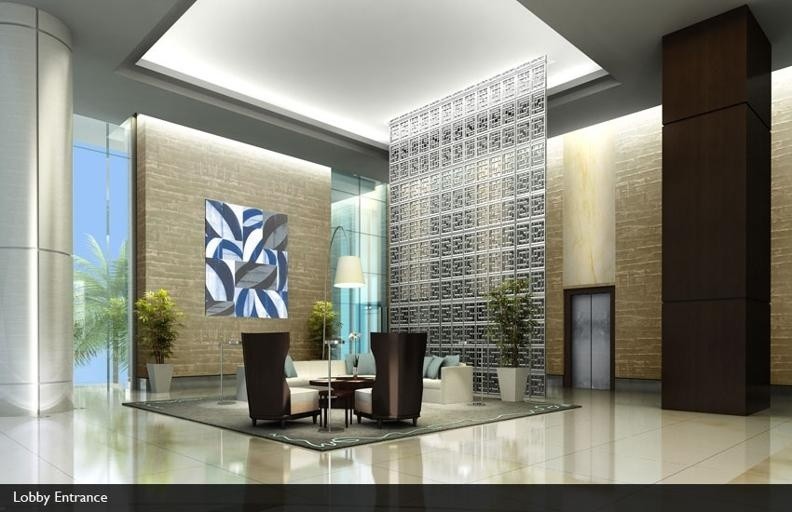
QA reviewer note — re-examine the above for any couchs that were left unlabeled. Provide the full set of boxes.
[236,360,347,403]
[355,353,474,404]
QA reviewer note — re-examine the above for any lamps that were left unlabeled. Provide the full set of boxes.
[322,226,364,358]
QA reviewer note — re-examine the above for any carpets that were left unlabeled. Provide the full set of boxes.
[121,391,582,451]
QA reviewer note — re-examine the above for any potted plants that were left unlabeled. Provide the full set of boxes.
[133,289,188,395]
[482,279,538,403]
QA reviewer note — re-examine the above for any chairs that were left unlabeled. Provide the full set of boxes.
[241,331,320,430]
[353,332,428,429]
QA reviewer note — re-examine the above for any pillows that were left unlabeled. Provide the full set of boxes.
[423,355,434,377]
[443,354,460,366]
[427,355,445,379]
[284,354,298,378]
[356,353,377,376]
